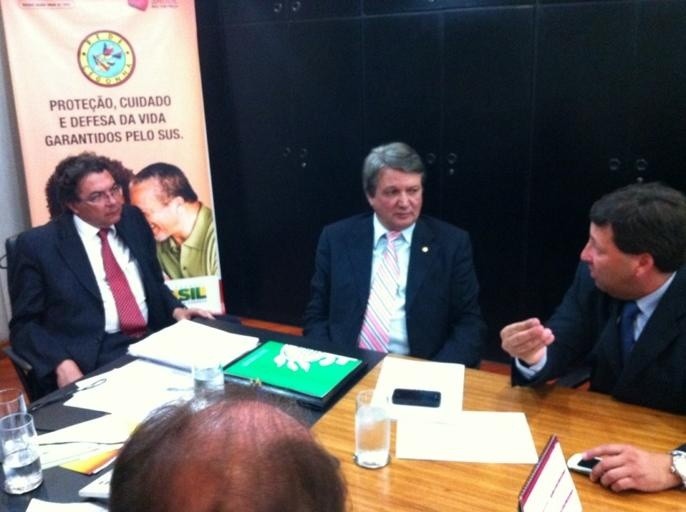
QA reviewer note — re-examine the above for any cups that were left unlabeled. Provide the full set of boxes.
[352,388,392,470]
[0,412,42,495]
[0,388,26,420]
[192,361,225,412]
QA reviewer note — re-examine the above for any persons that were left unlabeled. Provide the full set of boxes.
[499,181,686,493]
[302,142,486,369]
[107,383,349,512]
[129,162,219,279]
[9,152,217,402]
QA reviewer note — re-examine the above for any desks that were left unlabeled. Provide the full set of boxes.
[0,317,683,512]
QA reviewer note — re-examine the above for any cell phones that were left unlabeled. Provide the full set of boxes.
[392,388,441,407]
[566,452,602,475]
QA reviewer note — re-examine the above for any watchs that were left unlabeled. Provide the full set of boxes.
[669,450,686,489]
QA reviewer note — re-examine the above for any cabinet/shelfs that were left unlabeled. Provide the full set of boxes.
[525,0,686,324]
[215,0,366,330]
[358,0,540,372]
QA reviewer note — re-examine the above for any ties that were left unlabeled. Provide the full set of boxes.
[359,231,400,352]
[97,228,146,337]
[618,301,638,365]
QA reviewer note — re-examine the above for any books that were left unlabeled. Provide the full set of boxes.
[127,319,260,373]
[78,468,114,499]
